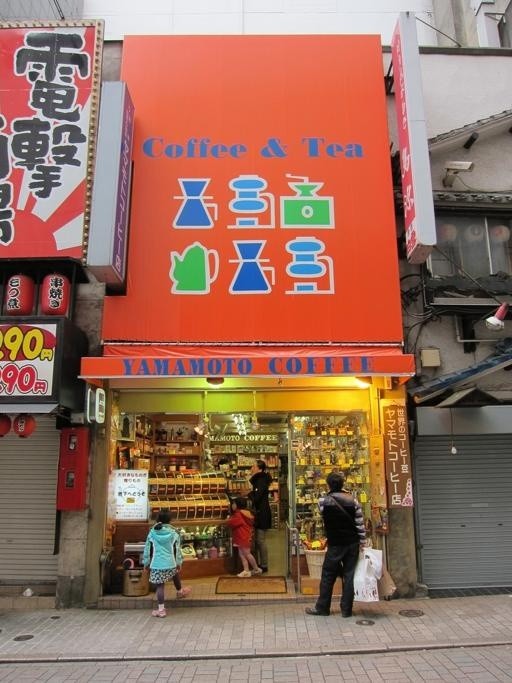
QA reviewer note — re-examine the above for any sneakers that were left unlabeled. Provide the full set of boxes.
[151,609,167,618]
[305,607,329,616]
[342,610,352,617]
[252,568,262,575]
[177,586,192,598]
[236,570,252,578]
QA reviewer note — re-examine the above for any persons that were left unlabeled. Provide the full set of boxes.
[304,471,366,617]
[243,460,272,573]
[224,497,263,578]
[142,507,193,617]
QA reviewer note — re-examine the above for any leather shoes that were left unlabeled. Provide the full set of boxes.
[259,567,269,572]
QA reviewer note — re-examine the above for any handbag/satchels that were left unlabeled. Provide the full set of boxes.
[353,549,379,602]
[363,538,383,580]
